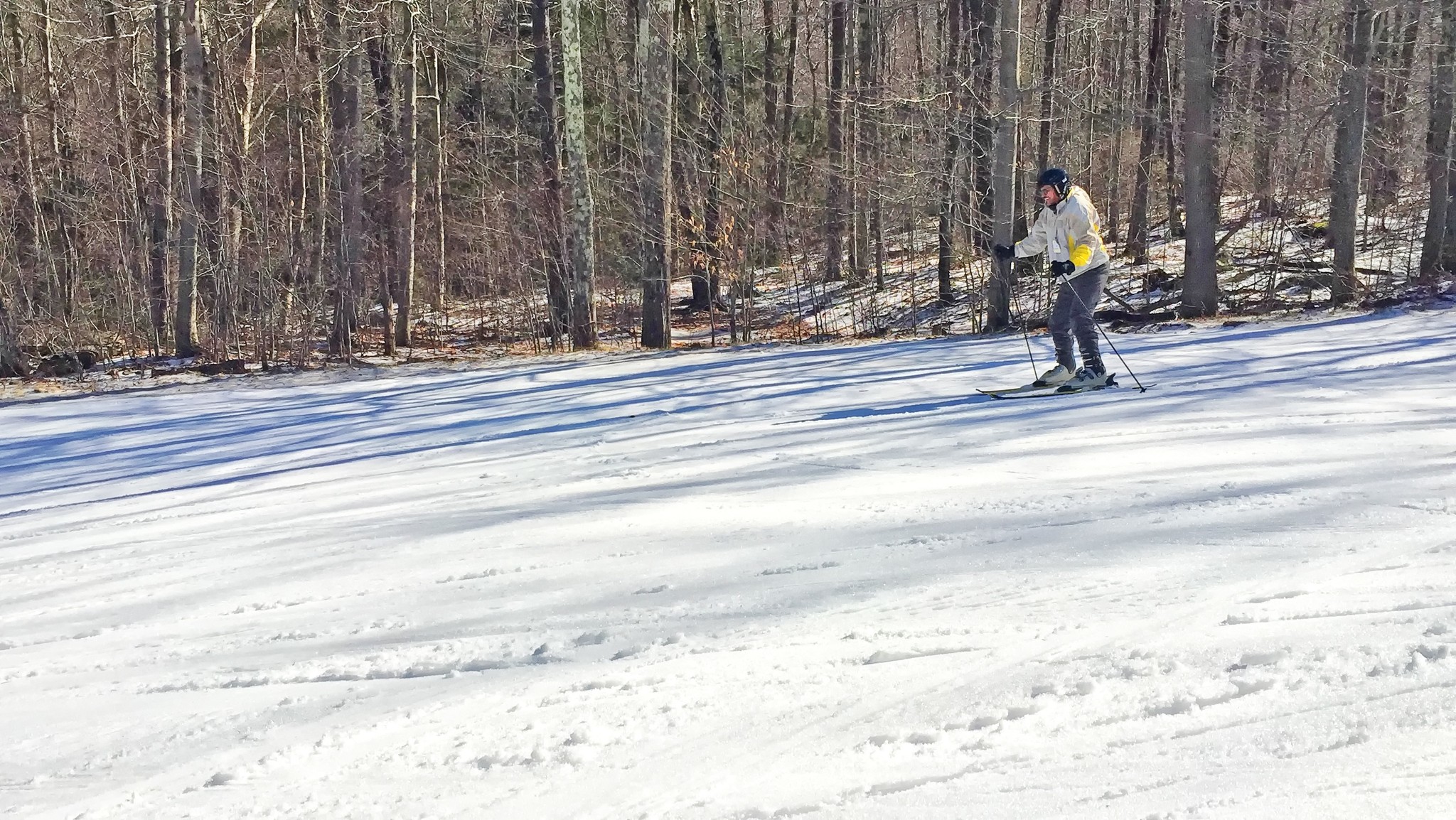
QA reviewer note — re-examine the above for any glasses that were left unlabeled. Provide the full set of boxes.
[1039,187,1049,195]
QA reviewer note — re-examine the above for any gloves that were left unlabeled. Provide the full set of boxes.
[1049,260,1074,277]
[992,244,1014,263]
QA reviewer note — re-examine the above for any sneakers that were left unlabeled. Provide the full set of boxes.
[1039,364,1077,381]
[1066,364,1106,386]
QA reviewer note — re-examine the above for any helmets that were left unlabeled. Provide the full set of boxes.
[1040,169,1070,199]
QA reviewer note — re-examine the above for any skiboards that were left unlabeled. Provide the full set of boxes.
[976,384,1157,400]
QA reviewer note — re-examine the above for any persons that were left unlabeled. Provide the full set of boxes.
[993,168,1112,390]
